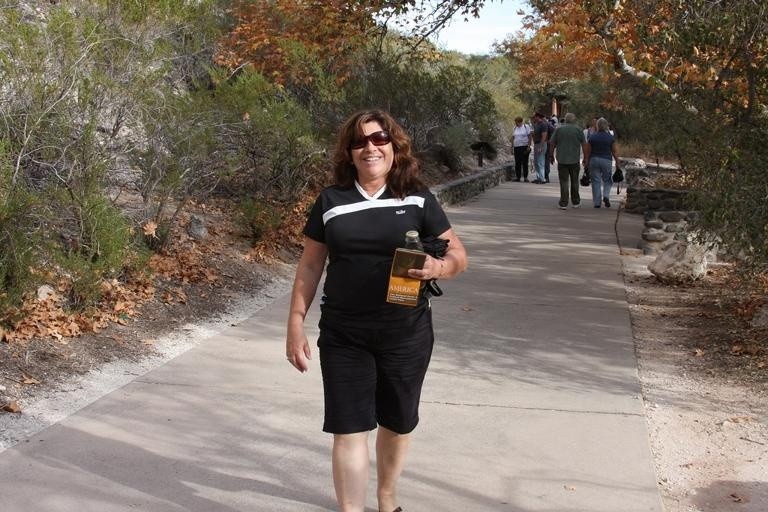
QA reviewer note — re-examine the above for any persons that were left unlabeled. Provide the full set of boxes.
[506,110,621,211]
[283,109,468,512]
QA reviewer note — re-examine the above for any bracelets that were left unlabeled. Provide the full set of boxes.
[437,258,444,278]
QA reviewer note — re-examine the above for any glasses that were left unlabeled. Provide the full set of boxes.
[351,129,393,149]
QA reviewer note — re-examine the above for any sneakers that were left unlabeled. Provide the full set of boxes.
[557,199,568,210]
[511,178,550,184]
[571,201,583,208]
[593,197,611,208]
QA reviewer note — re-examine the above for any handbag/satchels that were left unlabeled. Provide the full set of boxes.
[613,165,627,183]
[579,172,593,188]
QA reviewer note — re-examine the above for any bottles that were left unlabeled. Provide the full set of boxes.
[405,230,427,290]
